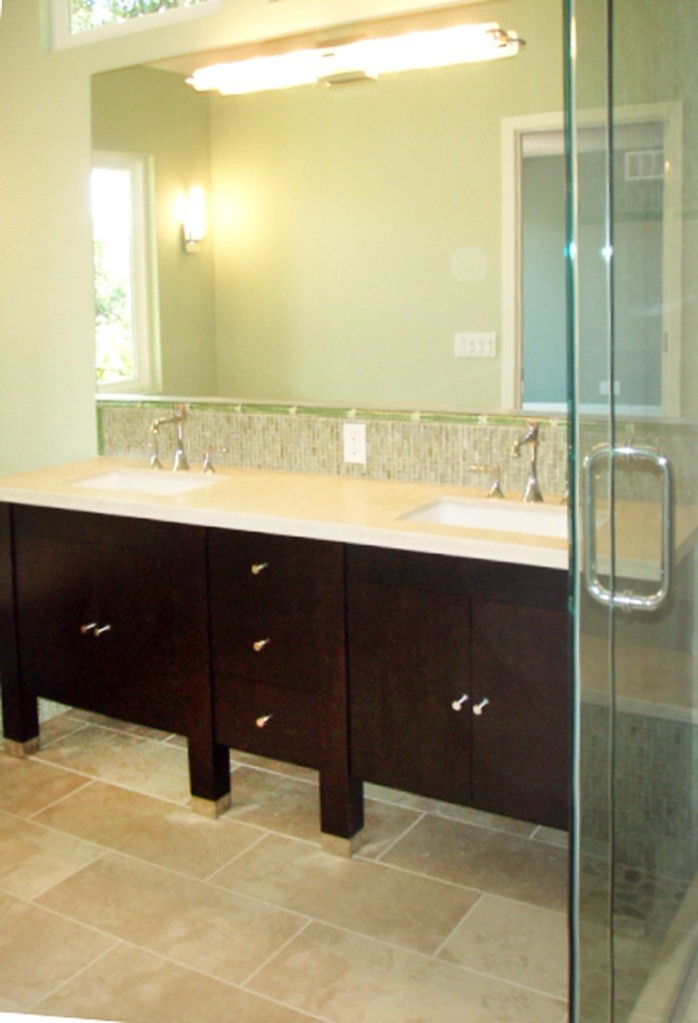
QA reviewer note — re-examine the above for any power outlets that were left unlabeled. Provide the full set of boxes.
[344,424,367,464]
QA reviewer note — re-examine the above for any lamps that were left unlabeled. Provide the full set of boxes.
[176,181,207,255]
[181,14,529,103]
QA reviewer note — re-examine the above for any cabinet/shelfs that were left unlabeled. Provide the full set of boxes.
[345,542,580,834]
[210,533,352,771]
[1,501,229,817]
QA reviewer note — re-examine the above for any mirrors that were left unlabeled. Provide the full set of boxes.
[91,0,698,427]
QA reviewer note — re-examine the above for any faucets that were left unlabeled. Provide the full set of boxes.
[508,418,546,505]
[149,403,191,472]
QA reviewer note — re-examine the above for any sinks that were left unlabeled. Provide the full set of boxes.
[390,495,618,542]
[68,465,230,497]
